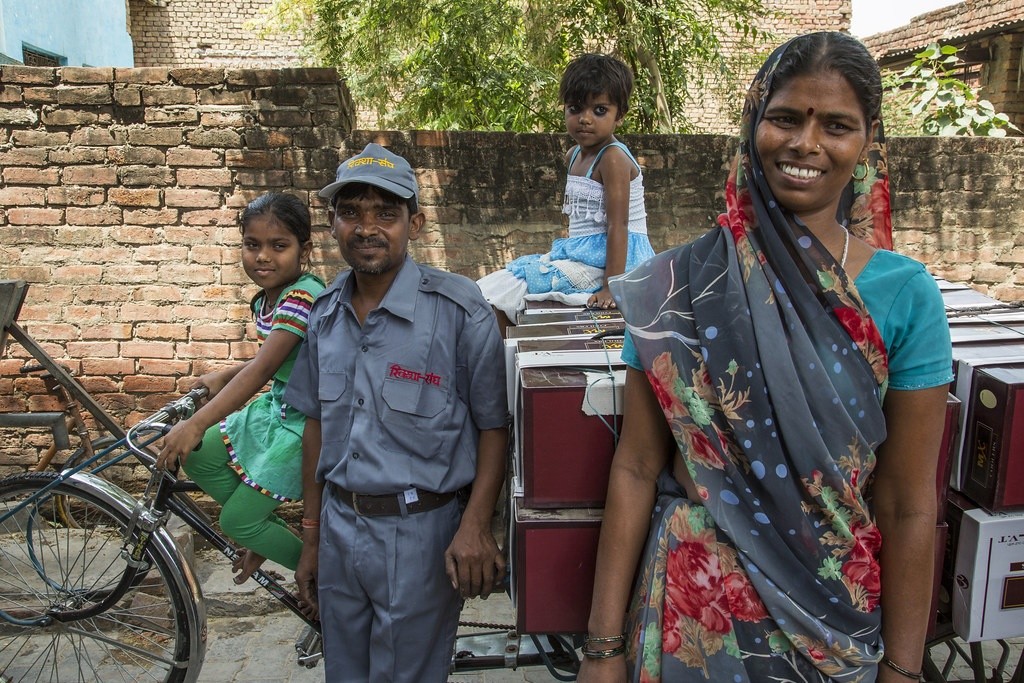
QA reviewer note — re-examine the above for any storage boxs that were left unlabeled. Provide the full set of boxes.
[503,274,1024,641]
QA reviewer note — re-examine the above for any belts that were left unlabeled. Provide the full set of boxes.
[329,480,457,517]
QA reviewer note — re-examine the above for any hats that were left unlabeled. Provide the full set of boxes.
[318,143,420,211]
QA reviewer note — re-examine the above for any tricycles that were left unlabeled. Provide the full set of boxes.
[1,273,1023,683]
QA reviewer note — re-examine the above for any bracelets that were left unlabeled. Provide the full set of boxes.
[882,656,924,680]
[583,628,624,644]
[581,643,626,658]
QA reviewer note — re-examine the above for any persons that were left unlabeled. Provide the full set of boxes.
[282,143,508,683]
[570,33,958,682]
[152,191,330,586]
[470,52,659,346]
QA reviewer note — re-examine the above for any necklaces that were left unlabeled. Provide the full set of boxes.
[829,222,853,288]
